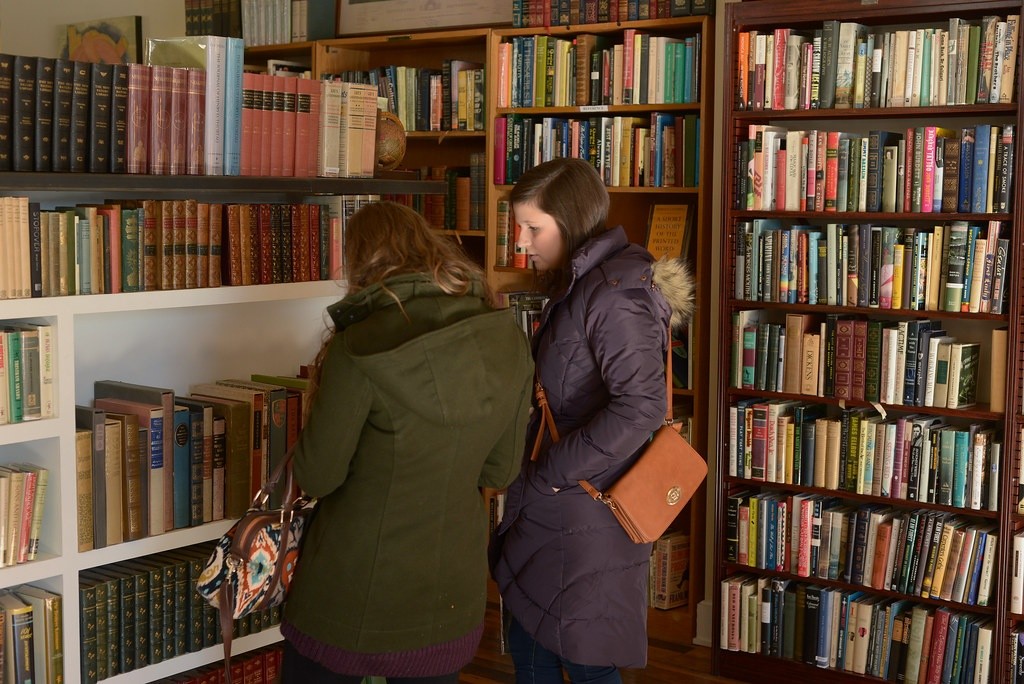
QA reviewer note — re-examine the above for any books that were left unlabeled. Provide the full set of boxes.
[0,0,1024,684]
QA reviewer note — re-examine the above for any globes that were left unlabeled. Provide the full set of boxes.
[376,109,418,179]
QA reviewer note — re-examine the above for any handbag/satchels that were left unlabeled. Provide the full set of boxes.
[196,444,316,619]
[606,426,709,544]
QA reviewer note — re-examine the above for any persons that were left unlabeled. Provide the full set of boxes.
[273,200,532,684]
[485,158,693,684]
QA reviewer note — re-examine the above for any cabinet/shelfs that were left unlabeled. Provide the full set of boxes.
[710,0,1024,684]
[315,27,492,282]
[0,167,449,684]
[488,14,714,658]
